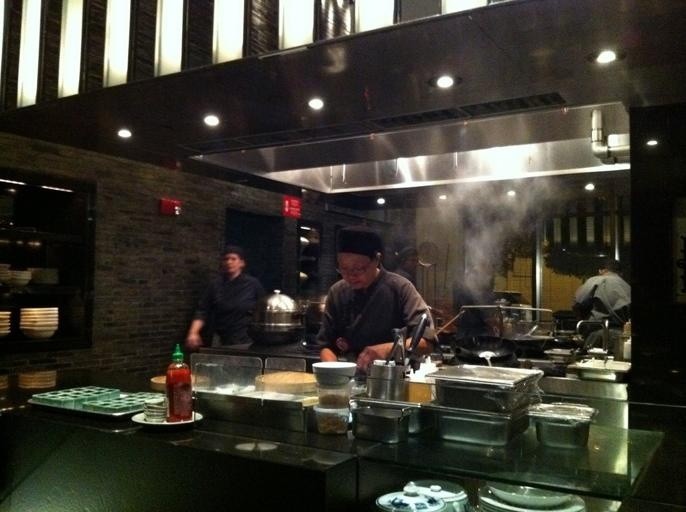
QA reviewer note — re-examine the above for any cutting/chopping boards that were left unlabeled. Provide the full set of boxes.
[147,374,211,392]
[255,370,319,393]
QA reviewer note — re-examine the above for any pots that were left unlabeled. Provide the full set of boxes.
[453,335,555,365]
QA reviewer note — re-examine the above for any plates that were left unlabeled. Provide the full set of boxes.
[475,472,587,512]
[0,306,60,339]
[0,263,60,287]
[131,409,206,426]
[1,368,57,389]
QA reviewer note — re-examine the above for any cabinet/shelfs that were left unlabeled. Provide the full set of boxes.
[1,170,100,350]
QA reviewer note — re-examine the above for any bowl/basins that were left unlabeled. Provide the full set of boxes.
[311,361,358,386]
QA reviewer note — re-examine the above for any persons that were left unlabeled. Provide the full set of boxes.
[318,227,438,369]
[452,242,495,337]
[576,259,631,329]
[395,244,419,287]
[186,243,266,350]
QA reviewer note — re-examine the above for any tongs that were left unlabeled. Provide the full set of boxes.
[383,334,407,380]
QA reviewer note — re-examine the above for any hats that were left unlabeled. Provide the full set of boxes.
[224,246,244,255]
[333,225,384,256]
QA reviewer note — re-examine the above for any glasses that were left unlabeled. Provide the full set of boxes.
[334,267,368,276]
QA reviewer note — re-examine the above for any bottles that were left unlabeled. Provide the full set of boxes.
[165,342,192,423]
